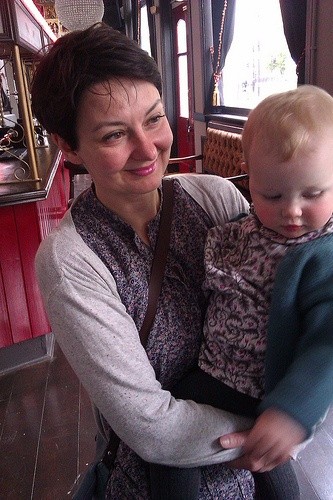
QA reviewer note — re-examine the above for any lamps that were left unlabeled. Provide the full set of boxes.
[54,0,104,32]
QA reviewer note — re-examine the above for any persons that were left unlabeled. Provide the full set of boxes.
[30,22,271,500]
[150,85,333,499]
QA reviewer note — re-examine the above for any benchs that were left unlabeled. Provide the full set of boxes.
[163,121,252,205]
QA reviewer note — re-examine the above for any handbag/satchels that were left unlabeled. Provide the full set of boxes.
[66,432,112,500]
